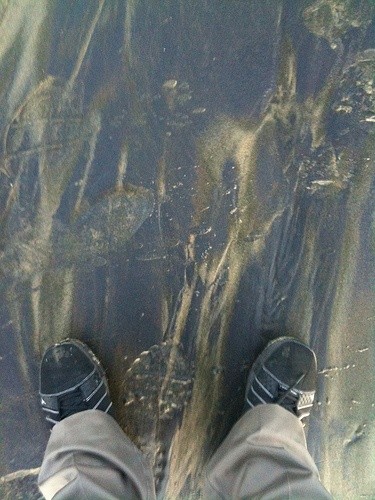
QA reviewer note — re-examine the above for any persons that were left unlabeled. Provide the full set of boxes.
[36,337,329,499]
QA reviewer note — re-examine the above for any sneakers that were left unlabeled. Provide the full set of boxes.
[38,338,116,434]
[240,336,318,439]
